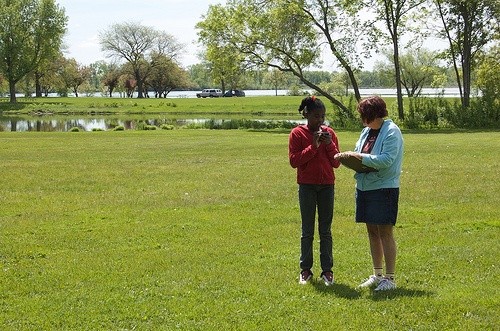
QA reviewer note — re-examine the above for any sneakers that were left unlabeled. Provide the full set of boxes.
[359,274,380,288]
[322,270,333,287]
[375,278,396,292]
[299,270,312,284]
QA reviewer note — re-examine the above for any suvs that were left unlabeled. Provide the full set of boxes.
[196,89,222,98]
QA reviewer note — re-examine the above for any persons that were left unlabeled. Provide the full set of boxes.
[334,95,404,289]
[288,97,340,286]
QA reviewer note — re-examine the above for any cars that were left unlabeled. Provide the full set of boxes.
[222,89,245,97]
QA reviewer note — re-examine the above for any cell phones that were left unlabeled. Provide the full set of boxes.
[321,132,329,138]
